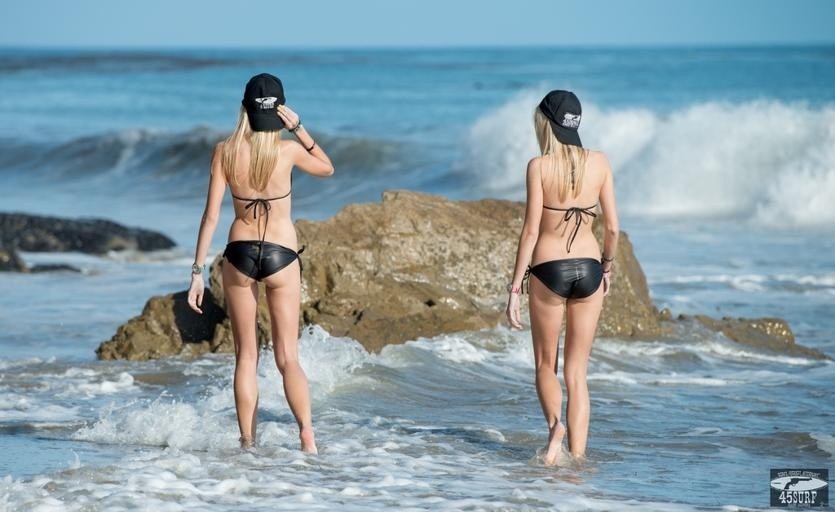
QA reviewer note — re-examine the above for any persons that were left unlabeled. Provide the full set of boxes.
[505,88,622,473]
[184,71,337,460]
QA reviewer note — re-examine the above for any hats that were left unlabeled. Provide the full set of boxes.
[241,72,287,132]
[538,90,583,148]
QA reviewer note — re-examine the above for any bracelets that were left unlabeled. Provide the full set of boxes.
[191,262,206,274]
[288,119,303,134]
[506,283,520,294]
[306,139,316,152]
[602,252,616,263]
[603,270,610,273]
